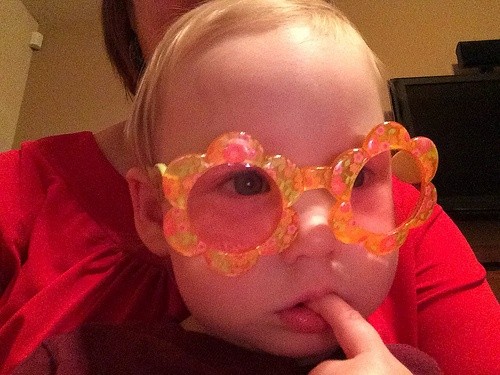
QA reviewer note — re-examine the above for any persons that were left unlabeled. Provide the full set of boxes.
[1,0,498,375]
[8,0,448,374]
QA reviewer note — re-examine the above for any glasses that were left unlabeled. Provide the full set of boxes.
[144,117,437,268]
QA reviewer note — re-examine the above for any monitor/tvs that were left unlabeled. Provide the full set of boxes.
[387,73,500,195]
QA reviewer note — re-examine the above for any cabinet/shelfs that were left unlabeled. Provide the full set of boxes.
[472,246,500,293]
